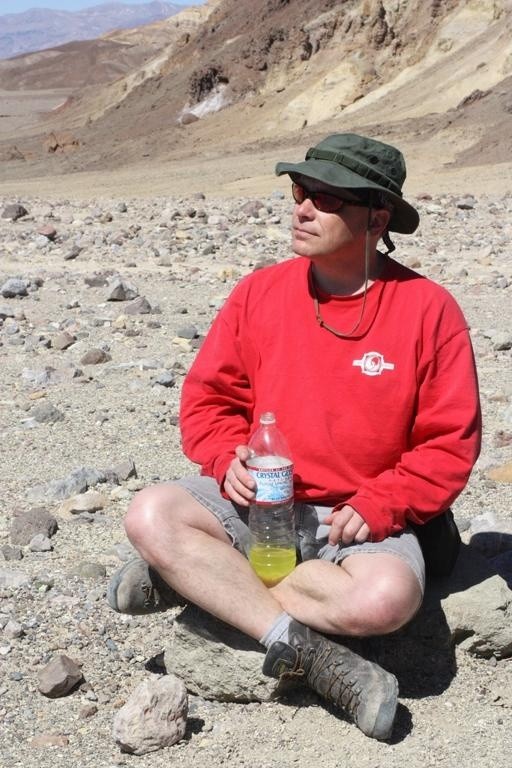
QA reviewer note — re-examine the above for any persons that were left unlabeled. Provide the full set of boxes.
[108,130,482,740]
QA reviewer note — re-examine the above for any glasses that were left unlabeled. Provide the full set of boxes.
[291,182,382,213]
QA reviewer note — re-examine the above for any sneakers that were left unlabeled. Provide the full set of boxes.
[262,621,399,740]
[108,558,187,616]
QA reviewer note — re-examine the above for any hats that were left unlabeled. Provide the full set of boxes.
[275,133,418,234]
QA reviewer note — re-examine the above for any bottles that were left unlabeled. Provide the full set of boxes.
[241,411,297,587]
